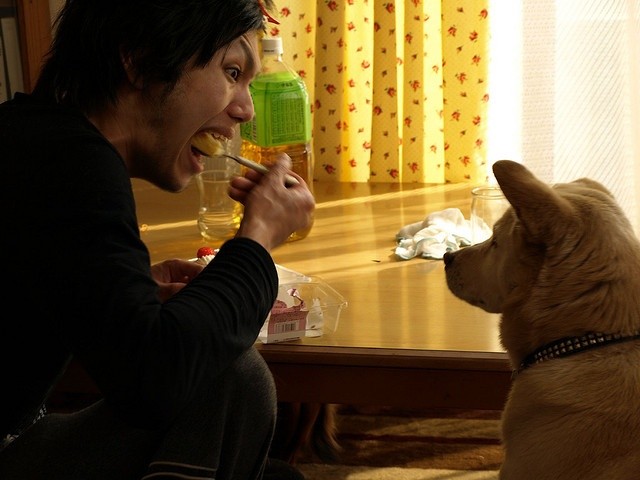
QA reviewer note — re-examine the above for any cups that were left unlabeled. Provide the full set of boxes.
[470,185,510,227]
[194,169,244,242]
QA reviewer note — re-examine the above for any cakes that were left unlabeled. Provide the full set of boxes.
[190,130,223,158]
[192,254,216,268]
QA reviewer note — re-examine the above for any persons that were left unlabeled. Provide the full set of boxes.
[0,1,316,480]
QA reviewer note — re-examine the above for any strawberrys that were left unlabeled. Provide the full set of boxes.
[197,247,218,258]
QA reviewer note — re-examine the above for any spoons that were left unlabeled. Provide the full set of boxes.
[189,133,299,187]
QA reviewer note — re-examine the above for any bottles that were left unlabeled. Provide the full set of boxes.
[240,36,316,245]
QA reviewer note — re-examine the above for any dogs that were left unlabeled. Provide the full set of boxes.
[442,160,640,480]
